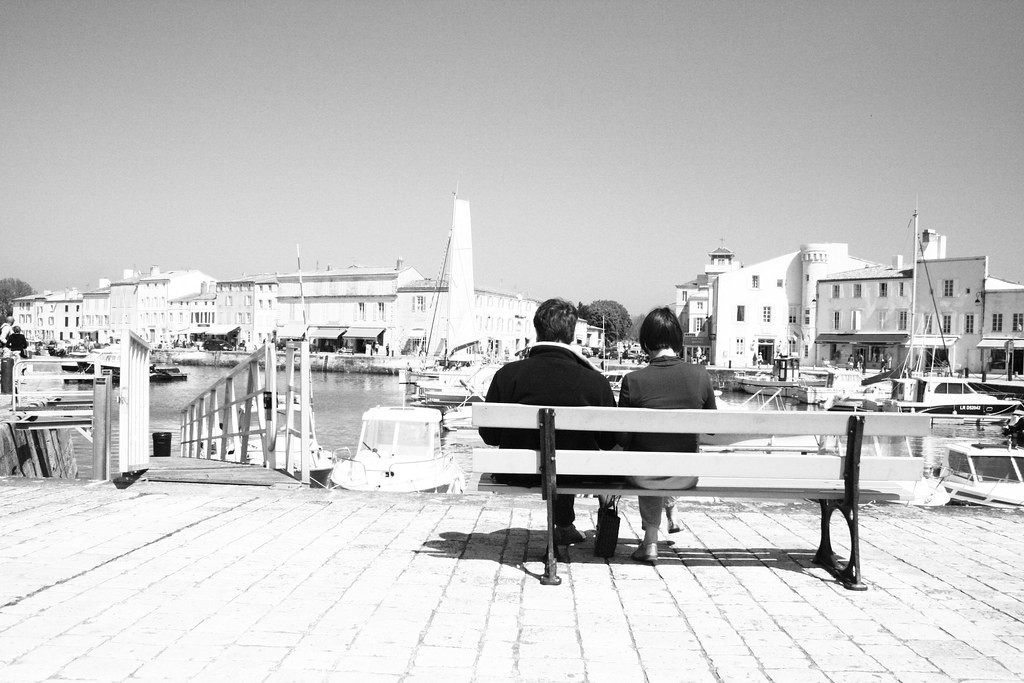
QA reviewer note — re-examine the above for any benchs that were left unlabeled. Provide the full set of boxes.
[471,402,934,592]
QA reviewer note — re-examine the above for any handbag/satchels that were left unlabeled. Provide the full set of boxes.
[594,500,620,558]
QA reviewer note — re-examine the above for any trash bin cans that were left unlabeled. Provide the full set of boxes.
[152,431,172,456]
[2,358,15,393]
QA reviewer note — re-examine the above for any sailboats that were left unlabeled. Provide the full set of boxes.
[832,205,1023,428]
[400,186,507,404]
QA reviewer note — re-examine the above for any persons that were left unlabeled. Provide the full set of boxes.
[271,337,390,356]
[504,347,509,362]
[755,349,893,375]
[631,350,651,366]
[157,339,247,351]
[0,316,15,357]
[618,309,718,560]
[478,298,620,546]
[6,326,29,357]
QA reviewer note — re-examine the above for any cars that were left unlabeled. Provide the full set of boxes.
[202,339,233,352]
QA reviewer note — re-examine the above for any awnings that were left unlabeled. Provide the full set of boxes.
[977,337,1024,350]
[204,325,240,335]
[305,326,349,339]
[905,334,961,348]
[178,327,209,334]
[342,326,385,339]
[277,325,310,338]
[813,334,908,345]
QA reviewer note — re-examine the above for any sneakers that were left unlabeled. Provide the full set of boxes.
[669,520,684,533]
[631,542,658,561]
[555,524,586,544]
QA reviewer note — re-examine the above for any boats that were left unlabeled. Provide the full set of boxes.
[4,353,106,438]
[602,368,639,403]
[145,363,186,383]
[932,436,1024,513]
[77,358,122,384]
[197,386,335,489]
[329,404,459,499]
[736,356,803,398]
[796,373,867,405]
